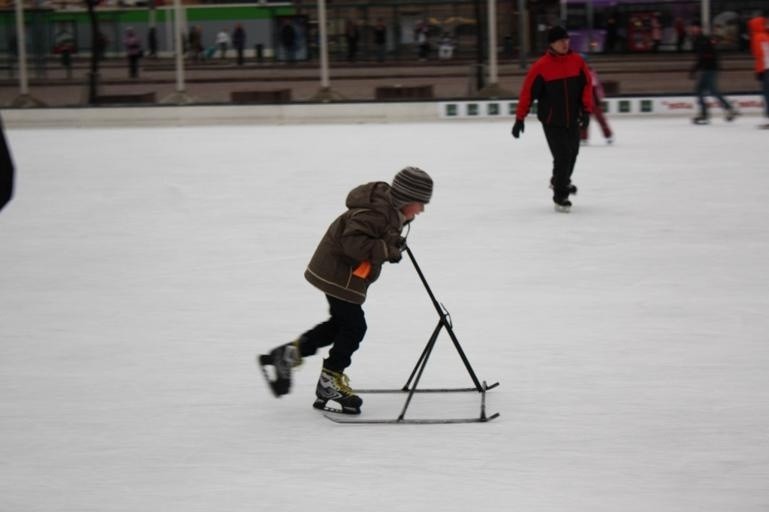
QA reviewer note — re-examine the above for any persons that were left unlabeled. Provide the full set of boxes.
[272,167,433,409]
[54,30,78,79]
[184,13,769,123]
[511,25,593,206]
[147,27,158,54]
[1,124,14,211]
[123,27,144,76]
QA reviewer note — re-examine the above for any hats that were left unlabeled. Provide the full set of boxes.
[391,166,433,204]
[547,25,571,45]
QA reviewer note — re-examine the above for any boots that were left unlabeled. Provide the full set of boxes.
[725,109,738,121]
[693,113,709,122]
[272,340,307,390]
[314,359,364,409]
[552,184,577,208]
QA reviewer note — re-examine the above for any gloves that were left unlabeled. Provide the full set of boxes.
[511,120,525,139]
[388,236,407,265]
